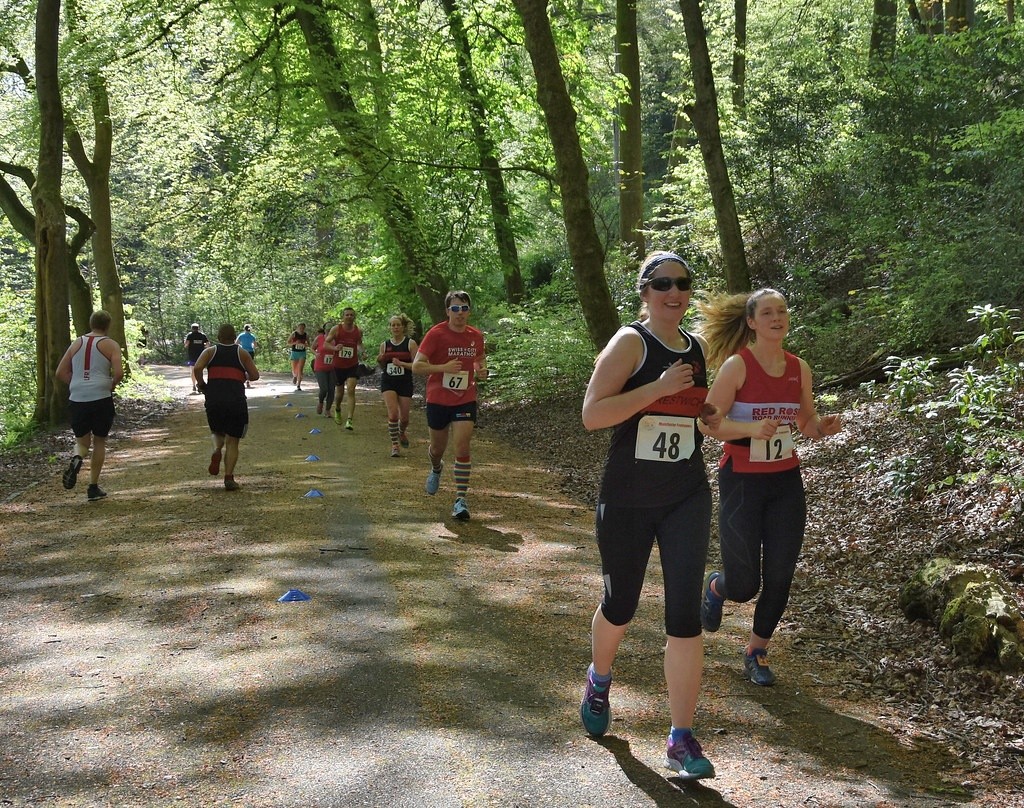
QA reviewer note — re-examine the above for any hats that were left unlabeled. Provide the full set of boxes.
[244,324,251,331]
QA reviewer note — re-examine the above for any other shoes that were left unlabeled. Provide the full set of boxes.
[209,452,222,475]
[297,387,301,391]
[193,387,197,390]
[317,403,323,413]
[323,410,331,418]
[247,381,251,387]
[87,486,107,500]
[292,377,296,384]
[62,455,83,489]
[224,480,243,491]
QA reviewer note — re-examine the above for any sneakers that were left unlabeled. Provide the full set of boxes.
[701,571,725,631]
[335,410,342,425]
[425,470,442,494]
[581,662,611,738]
[391,447,400,456]
[399,435,409,449]
[345,420,352,429]
[664,733,715,780]
[451,500,470,520]
[743,648,777,686]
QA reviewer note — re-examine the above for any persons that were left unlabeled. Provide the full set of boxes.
[693,289,842,686]
[412,291,489,519]
[56,310,124,501]
[193,324,259,491]
[235,324,257,388]
[317,328,325,337]
[377,313,418,457]
[184,323,210,395]
[311,322,335,418]
[581,250,722,781]
[288,323,311,391]
[323,308,368,431]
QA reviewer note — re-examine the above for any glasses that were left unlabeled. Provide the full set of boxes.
[640,276,692,291]
[447,305,470,312]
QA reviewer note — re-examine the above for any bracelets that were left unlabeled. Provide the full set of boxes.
[699,415,707,426]
[362,352,367,355]
[487,370,489,376]
